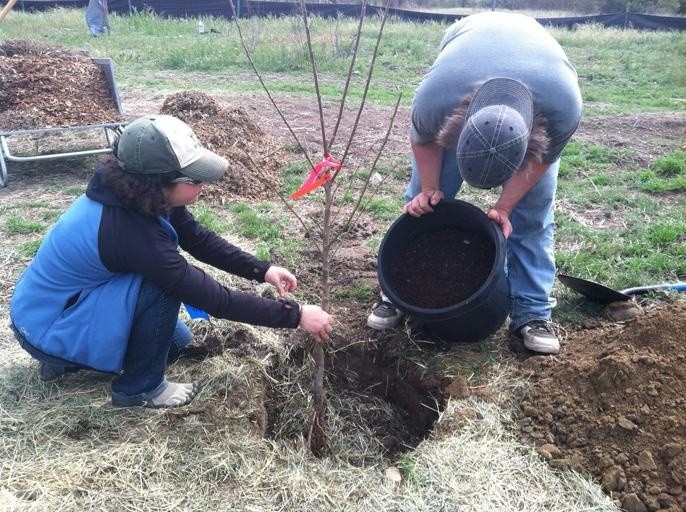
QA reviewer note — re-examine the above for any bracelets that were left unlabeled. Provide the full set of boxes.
[298,303,303,318]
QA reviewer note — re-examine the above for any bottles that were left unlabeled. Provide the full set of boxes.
[199,20,204,34]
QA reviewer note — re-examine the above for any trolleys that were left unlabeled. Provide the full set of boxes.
[0,53,129,185]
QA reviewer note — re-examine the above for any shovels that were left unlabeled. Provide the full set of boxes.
[558,274,686,301]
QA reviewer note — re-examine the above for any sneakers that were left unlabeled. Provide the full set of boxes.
[39,363,80,382]
[365,290,406,331]
[110,381,200,408]
[518,320,562,355]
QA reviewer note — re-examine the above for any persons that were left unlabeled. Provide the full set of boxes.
[366,9,583,353]
[11,112,331,409]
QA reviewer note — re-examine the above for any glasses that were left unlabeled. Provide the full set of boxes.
[170,176,204,186]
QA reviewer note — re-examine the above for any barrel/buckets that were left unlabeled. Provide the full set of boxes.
[376,200,512,341]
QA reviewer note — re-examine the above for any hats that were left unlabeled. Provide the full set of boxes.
[111,114,230,182]
[455,77,535,191]
